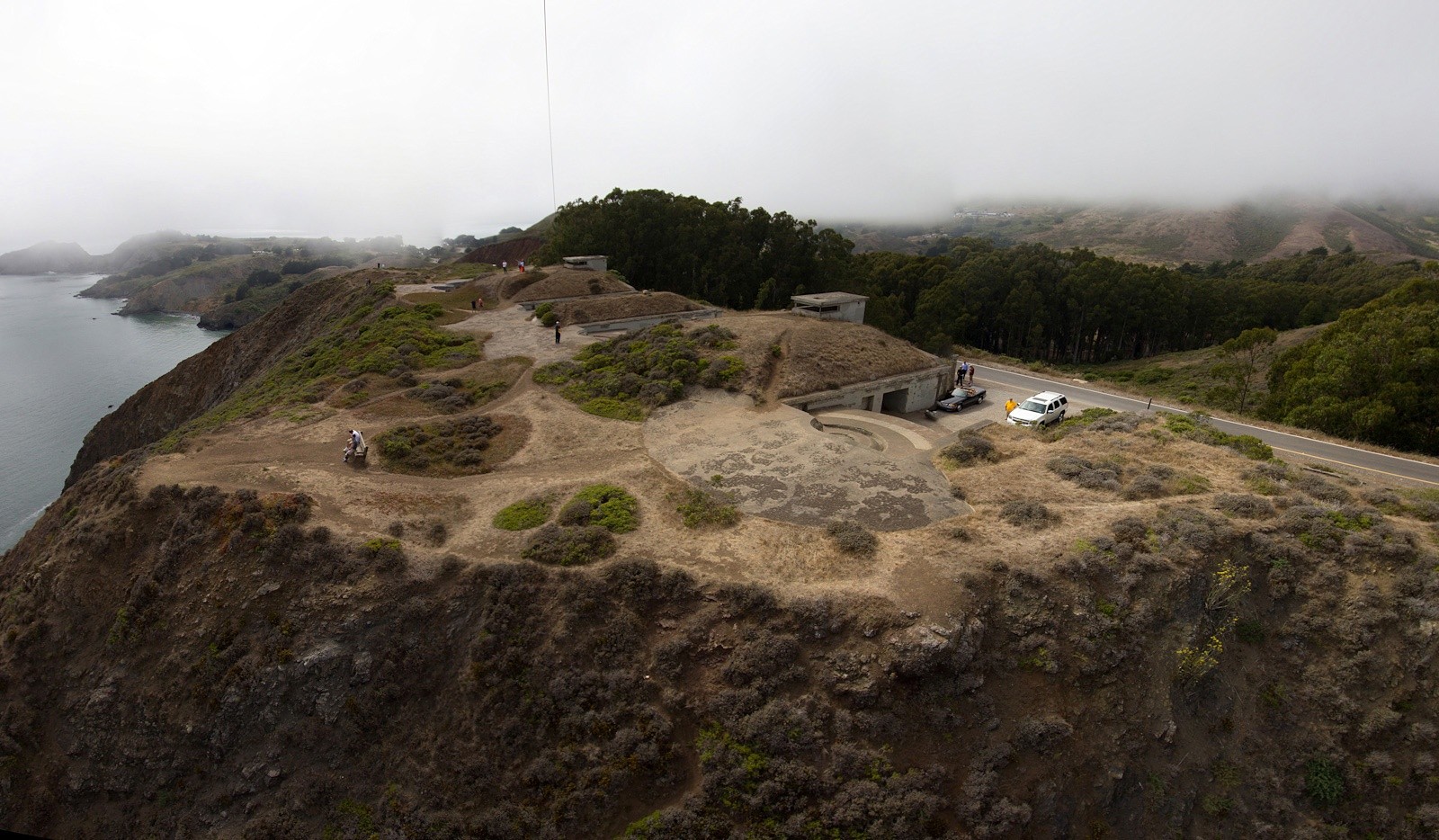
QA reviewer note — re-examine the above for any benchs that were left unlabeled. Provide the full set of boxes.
[353,431,368,461]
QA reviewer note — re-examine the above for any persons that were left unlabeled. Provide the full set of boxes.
[343,429,360,461]
[554,320,561,344]
[1004,398,1018,419]
[956,361,974,387]
[366,278,371,287]
[518,258,525,272]
[471,296,483,313]
[502,260,508,274]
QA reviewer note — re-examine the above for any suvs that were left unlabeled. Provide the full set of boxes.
[1007,391,1068,430]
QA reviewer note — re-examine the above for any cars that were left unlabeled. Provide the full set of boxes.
[935,386,987,412]
